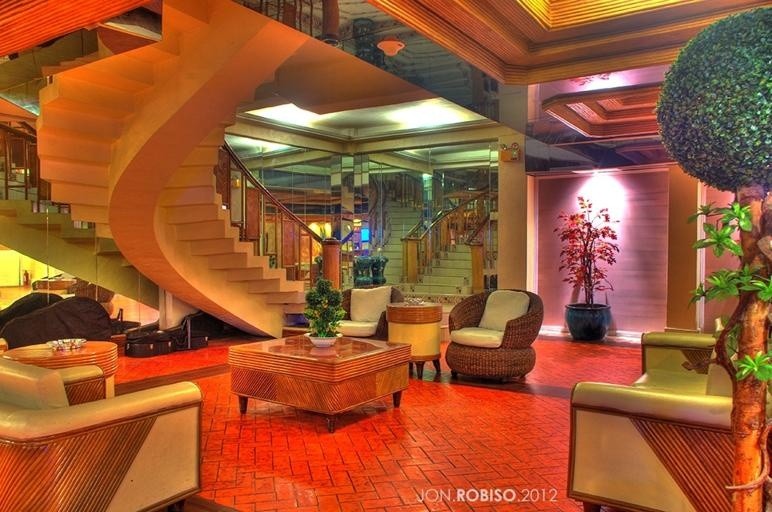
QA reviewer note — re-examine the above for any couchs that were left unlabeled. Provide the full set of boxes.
[0,333,203,511]
[565,330,770,511]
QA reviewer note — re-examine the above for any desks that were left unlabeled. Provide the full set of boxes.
[1,340,119,397]
[386,300,443,382]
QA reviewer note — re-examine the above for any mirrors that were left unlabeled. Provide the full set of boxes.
[245,140,498,328]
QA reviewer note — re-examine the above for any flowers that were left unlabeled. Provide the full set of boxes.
[553,195,621,308]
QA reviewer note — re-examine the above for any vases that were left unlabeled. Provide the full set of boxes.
[565,304,610,343]
[353,254,373,287]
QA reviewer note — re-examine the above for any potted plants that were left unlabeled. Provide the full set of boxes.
[300,279,347,347]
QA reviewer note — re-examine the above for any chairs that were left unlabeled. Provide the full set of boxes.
[335,284,403,341]
[445,288,544,385]
